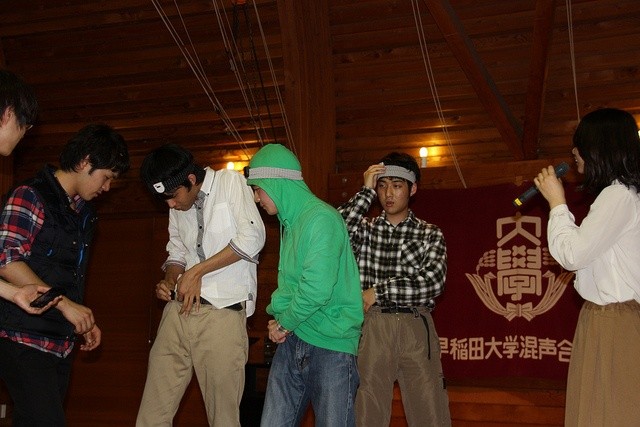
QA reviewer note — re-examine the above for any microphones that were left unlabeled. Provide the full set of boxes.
[511,161,570,207]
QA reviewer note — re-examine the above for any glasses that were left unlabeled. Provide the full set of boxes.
[13,109,34,131]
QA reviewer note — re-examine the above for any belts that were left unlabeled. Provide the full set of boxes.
[380,307,431,360]
[170,290,243,311]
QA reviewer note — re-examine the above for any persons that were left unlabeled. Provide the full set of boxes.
[136,147,266,427]
[0,124,130,427]
[0,71,63,314]
[244,143,364,427]
[534,107,640,427]
[337,152,451,427]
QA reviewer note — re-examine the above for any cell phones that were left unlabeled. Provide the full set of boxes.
[30,286,65,307]
